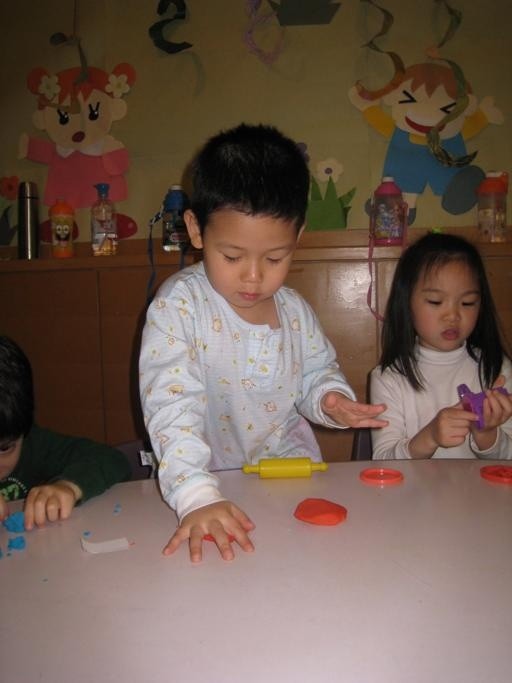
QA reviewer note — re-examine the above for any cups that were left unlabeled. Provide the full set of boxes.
[48,194,75,260]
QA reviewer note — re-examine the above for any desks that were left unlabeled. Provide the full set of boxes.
[0,466,511,683]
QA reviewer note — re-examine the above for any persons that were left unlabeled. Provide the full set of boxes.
[138,122,387,563]
[370,231,511,460]
[1,334,130,532]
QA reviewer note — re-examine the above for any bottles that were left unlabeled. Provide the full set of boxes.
[89,180,121,258]
[474,170,509,244]
[161,183,192,253]
[16,178,40,259]
[366,176,408,247]
